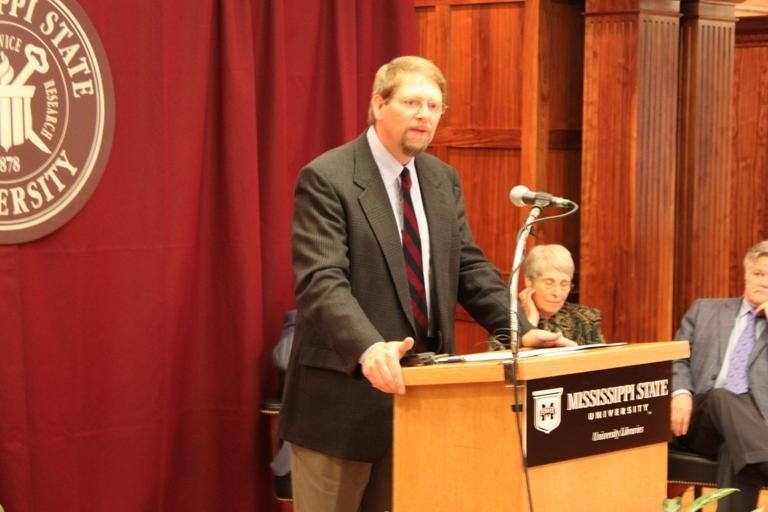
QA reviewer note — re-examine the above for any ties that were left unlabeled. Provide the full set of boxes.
[725,308,759,394]
[398,166,429,355]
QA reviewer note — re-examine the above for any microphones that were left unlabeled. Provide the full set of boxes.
[508,182,571,208]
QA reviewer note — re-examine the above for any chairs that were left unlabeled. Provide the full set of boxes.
[666,435,720,511]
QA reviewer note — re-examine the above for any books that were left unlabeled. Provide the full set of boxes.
[434,346,544,364]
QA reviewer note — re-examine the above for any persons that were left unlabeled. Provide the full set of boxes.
[665,238,768,512]
[271,52,583,512]
[483,243,607,350]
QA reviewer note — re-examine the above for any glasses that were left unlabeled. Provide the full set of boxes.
[384,96,448,116]
